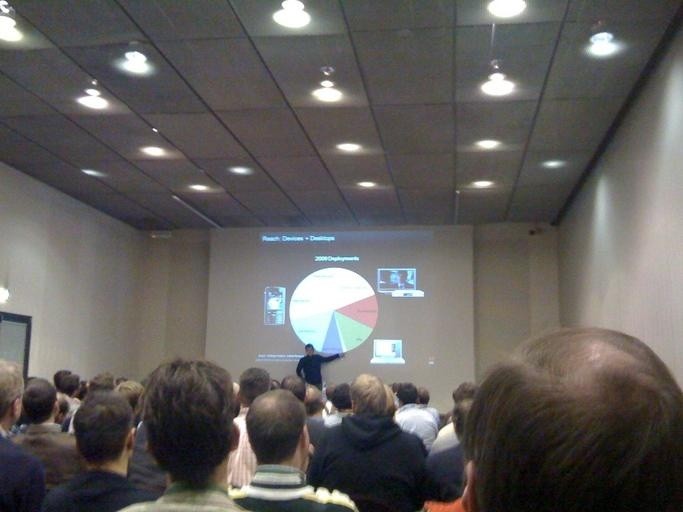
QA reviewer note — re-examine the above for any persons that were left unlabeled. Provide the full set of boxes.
[268,292,281,308]
[388,343,398,357]
[2,360,478,510]
[294,344,345,389]
[460,325,683,511]
[380,270,396,288]
[394,271,413,288]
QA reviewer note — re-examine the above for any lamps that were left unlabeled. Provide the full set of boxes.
[316,66,338,89]
[81,78,104,97]
[585,18,617,46]
[122,38,151,68]
[0,2,19,31]
[487,57,507,82]
